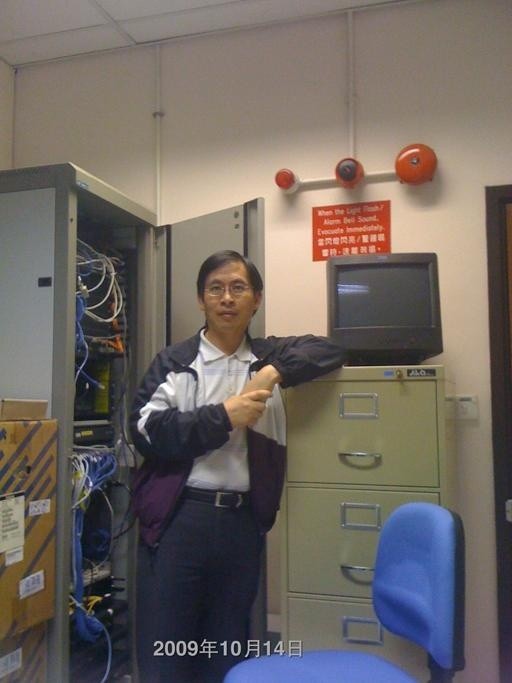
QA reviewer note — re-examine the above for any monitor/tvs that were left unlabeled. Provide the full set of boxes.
[327,253,443,366]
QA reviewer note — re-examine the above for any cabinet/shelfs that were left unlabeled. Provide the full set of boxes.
[275,360,459,676]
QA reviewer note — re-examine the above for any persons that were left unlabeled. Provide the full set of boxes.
[128,247,346,683]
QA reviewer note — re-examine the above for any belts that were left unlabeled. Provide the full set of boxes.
[183,488,250,508]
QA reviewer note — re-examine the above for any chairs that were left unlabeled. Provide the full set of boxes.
[217,501,466,683]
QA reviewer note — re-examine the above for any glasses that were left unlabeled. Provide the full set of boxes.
[202,283,253,296]
